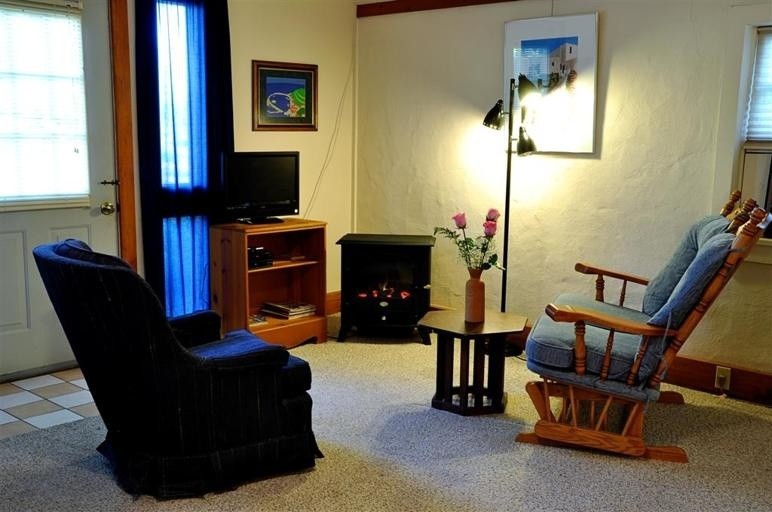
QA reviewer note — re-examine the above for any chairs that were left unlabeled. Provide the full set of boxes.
[515,185,768,464]
[32,235,313,499]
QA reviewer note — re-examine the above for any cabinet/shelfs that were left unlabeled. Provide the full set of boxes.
[210,217,330,347]
[332,233,437,344]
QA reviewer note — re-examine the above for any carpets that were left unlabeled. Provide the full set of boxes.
[1,330,772,510]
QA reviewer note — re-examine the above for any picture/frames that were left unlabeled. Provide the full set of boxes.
[252,59,319,130]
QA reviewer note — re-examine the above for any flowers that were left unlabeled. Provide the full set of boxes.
[432,207,505,281]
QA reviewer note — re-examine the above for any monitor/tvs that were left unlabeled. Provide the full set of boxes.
[221,151,299,225]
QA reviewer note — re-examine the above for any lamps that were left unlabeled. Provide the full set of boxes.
[482,74,539,313]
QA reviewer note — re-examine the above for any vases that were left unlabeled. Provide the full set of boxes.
[464,266,485,324]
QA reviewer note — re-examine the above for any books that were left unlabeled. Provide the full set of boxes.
[260,299,317,320]
[249,315,268,327]
[274,254,305,261]
[267,259,291,265]
